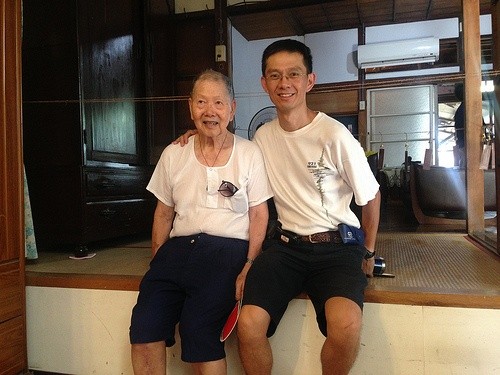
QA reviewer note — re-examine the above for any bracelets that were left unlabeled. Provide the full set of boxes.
[362,246,375,259]
[246,258,254,264]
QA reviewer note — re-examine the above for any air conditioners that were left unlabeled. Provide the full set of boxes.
[358,36,439,69]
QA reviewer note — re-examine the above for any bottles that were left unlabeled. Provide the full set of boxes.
[374,257,386,275]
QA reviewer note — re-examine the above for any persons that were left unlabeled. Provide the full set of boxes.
[174,39,380,375]
[129,69,275,375]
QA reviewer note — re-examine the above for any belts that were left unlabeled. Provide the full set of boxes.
[276,226,343,243]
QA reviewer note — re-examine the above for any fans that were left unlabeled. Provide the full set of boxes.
[248,106,278,140]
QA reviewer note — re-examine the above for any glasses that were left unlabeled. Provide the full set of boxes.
[218,180,238,197]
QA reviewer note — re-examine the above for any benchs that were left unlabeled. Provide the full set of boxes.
[410,163,497,232]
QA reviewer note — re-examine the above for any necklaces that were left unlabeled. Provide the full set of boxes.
[199,131,229,167]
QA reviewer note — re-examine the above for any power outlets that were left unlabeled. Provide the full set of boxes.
[216,45,226,61]
